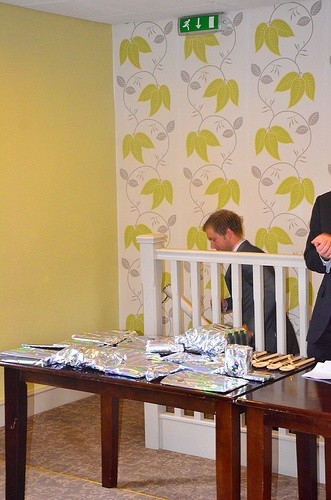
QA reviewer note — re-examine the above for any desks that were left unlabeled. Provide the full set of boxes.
[1,341,316,500]
[234,367,331,500]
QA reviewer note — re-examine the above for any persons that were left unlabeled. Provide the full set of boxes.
[304,190,331,363]
[204,209,300,356]
